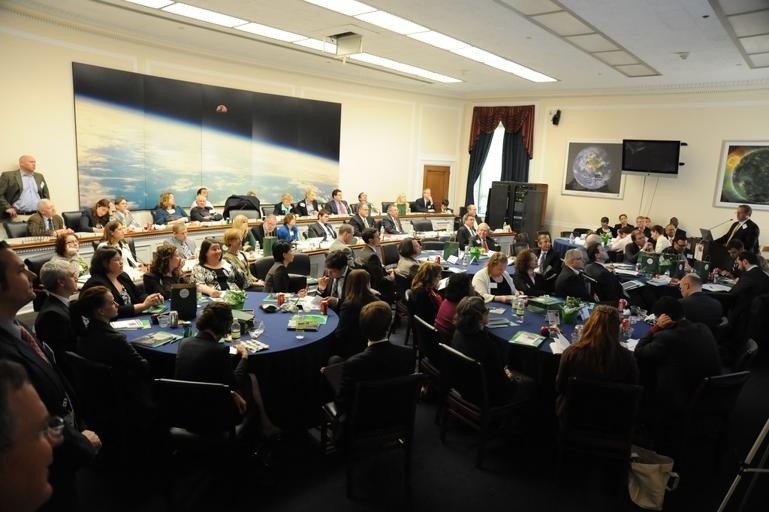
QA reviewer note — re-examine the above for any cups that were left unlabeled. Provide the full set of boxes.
[623,326,634,340]
[570,333,581,343]
[630,306,646,320]
[157,316,169,328]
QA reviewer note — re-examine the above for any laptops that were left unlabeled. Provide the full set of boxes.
[700,229,713,242]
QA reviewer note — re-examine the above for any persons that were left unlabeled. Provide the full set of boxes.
[0,187,431,512]
[410,188,769,511]
[0,154,51,223]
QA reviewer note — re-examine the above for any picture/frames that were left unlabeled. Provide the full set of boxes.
[561,139,625,200]
[713,140,769,211]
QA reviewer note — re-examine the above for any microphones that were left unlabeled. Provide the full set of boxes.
[699,219,733,243]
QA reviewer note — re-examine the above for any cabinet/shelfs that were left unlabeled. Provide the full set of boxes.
[489,181,548,244]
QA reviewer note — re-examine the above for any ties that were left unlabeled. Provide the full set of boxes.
[338,200,347,214]
[725,221,742,248]
[324,222,337,240]
[332,279,341,298]
[46,217,55,233]
[11,319,70,415]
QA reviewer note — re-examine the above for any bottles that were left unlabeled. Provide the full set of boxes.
[255,240,261,258]
[462,256,468,270]
[295,309,305,340]
[511,292,525,324]
[231,317,241,345]
[610,261,615,274]
[569,232,574,244]
[380,226,385,242]
[122,218,126,228]
[713,271,719,284]
[503,221,511,233]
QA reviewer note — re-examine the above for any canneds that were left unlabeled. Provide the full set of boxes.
[540,326,548,335]
[435,256,440,264]
[622,319,630,332]
[320,300,328,315]
[169,311,179,328]
[183,321,192,338]
[278,294,285,306]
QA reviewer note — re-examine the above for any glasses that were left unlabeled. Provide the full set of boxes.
[18,416,65,441]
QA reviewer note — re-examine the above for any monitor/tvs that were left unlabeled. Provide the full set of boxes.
[622,139,680,178]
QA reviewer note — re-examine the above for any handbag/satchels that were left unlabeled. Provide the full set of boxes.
[627,443,680,512]
